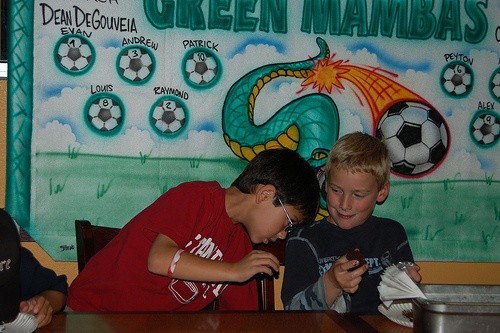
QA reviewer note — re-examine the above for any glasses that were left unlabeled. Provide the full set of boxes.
[279,198,299,233]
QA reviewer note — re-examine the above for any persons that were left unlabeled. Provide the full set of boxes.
[64,146,321,312]
[280,132,422,314]
[0,208,69,330]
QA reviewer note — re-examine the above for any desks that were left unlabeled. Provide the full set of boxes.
[0,309,413,333]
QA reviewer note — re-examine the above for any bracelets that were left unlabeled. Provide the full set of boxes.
[168,249,185,278]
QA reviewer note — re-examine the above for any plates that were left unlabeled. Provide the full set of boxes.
[0,312,38,333]
[378,303,413,328]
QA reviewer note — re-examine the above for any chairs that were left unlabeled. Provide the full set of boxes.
[74,220,124,274]
[257,237,288,310]
